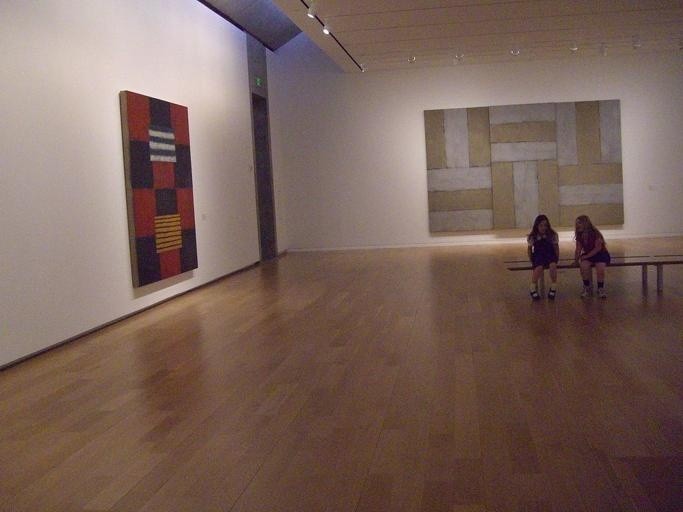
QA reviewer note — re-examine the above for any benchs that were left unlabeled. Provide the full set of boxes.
[503,250,683,297]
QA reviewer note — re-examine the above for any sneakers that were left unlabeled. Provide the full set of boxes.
[530,291,539,300]
[548,288,556,299]
[598,287,608,298]
[580,288,591,297]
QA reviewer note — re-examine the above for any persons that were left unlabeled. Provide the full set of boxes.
[569,215,610,299]
[525,214,559,300]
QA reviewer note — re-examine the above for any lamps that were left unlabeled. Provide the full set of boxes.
[307,0,332,35]
